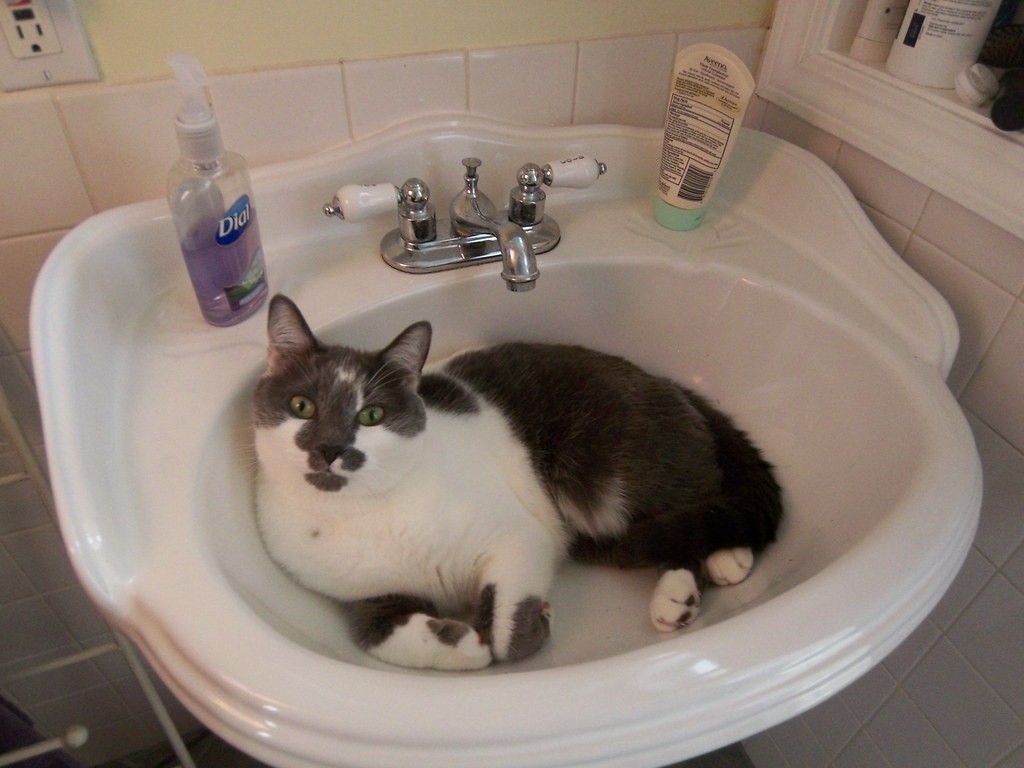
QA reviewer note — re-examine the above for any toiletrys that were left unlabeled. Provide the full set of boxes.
[166,49,272,332]
[849,0,1003,90]
[652,41,757,232]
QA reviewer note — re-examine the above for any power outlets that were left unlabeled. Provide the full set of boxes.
[0,0,102,93]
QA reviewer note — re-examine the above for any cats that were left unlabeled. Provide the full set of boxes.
[250,293,783,673]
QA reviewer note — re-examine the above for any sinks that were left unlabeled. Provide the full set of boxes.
[24,103,986,768]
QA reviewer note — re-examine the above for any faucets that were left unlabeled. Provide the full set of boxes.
[448,155,542,294]
